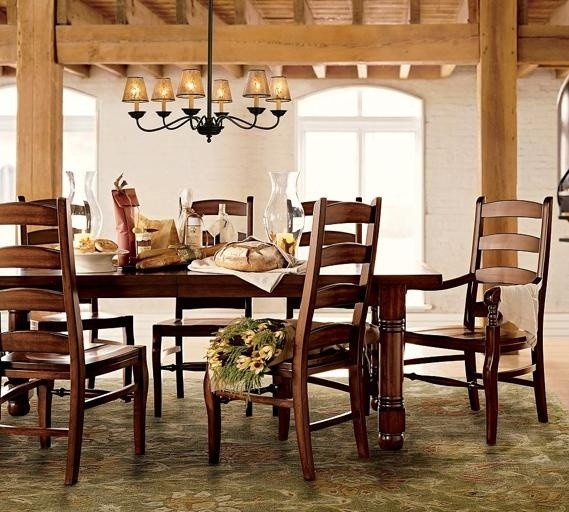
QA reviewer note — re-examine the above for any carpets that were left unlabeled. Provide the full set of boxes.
[0,372,567,512]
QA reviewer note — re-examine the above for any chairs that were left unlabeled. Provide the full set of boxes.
[15,192,136,405]
[379,195,555,445]
[145,195,260,416]
[205,194,382,477]
[274,195,378,422]
[0,196,150,484]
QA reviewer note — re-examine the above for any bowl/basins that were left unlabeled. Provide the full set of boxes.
[74,250,117,273]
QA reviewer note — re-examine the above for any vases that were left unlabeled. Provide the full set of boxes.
[65,169,103,243]
[267,170,307,259]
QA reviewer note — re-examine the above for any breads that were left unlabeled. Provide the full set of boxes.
[214,240,285,273]
[94,239,118,252]
[135,242,227,269]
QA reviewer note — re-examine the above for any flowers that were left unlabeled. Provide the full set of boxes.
[208,315,286,394]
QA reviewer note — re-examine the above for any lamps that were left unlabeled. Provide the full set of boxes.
[120,1,296,145]
